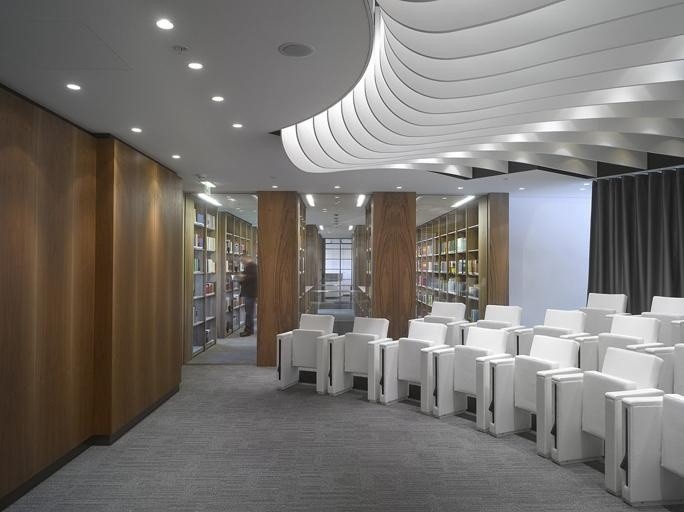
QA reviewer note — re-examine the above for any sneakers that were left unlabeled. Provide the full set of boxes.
[240,331,250,336]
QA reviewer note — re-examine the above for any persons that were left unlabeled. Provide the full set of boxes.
[238,254,257,337]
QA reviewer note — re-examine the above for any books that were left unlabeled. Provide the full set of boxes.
[193,208,216,344]
[225,239,247,332]
[415,235,479,321]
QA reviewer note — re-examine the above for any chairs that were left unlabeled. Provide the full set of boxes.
[489,335,582,459]
[327,316,393,403]
[379,322,450,415]
[431,320,526,433]
[551,347,664,496]
[622,394,684,507]
[408,292,684,394]
[275,314,338,394]
